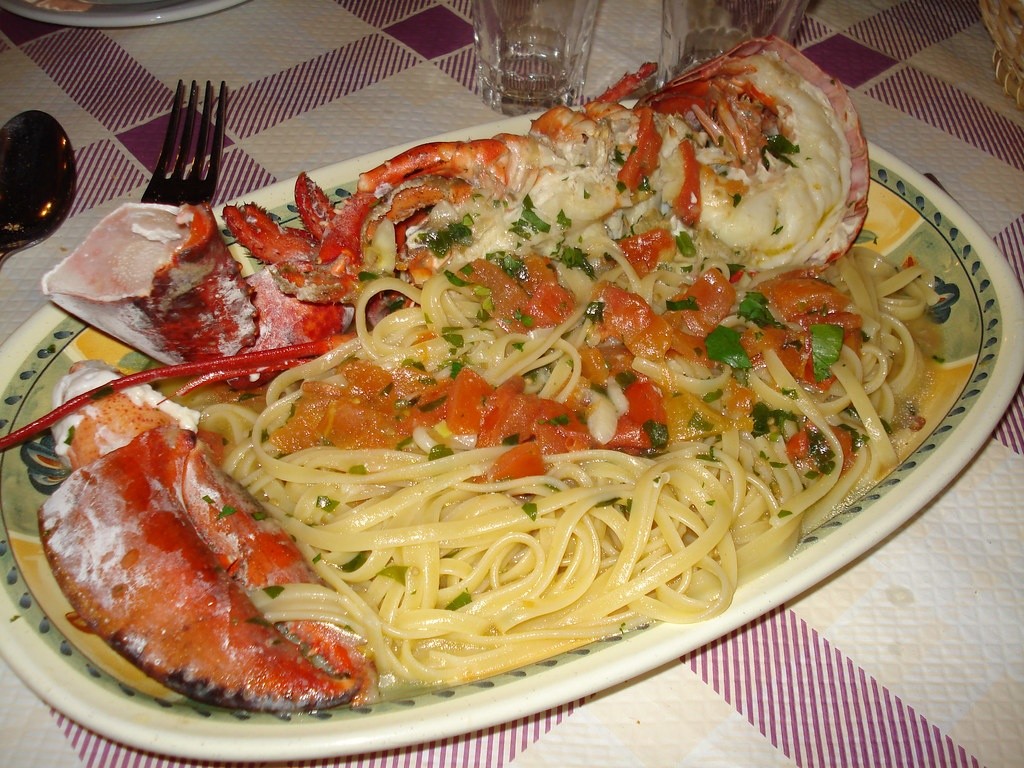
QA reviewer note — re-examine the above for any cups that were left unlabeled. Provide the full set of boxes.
[471,0,599,117]
[652,0,811,89]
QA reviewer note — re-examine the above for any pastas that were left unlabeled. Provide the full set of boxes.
[198,167,928,695]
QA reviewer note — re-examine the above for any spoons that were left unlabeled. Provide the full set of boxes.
[0,110,77,273]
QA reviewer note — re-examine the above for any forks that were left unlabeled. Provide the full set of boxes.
[140,79,228,207]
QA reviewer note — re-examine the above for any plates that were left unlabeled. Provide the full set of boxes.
[0,0,251,27]
[0,98,1024,763]
